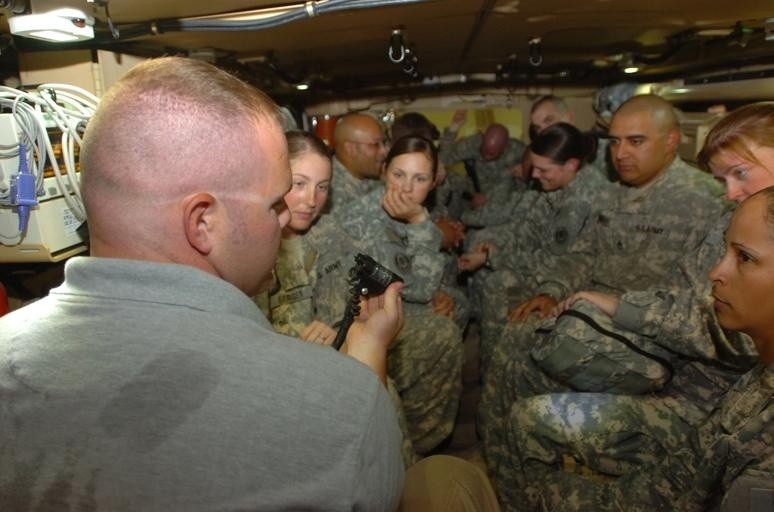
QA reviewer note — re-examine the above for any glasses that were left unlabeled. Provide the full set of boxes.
[344,135,387,149]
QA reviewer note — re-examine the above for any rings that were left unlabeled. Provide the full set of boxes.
[319,334,326,342]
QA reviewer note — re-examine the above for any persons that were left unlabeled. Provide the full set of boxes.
[524,184,773,510]
[328,133,472,337]
[248,129,464,473]
[329,113,457,285]
[389,111,441,150]
[0,56,501,512]
[456,121,613,382]
[442,124,528,202]
[463,95,608,348]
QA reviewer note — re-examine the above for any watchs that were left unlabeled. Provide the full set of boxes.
[485,253,494,269]
